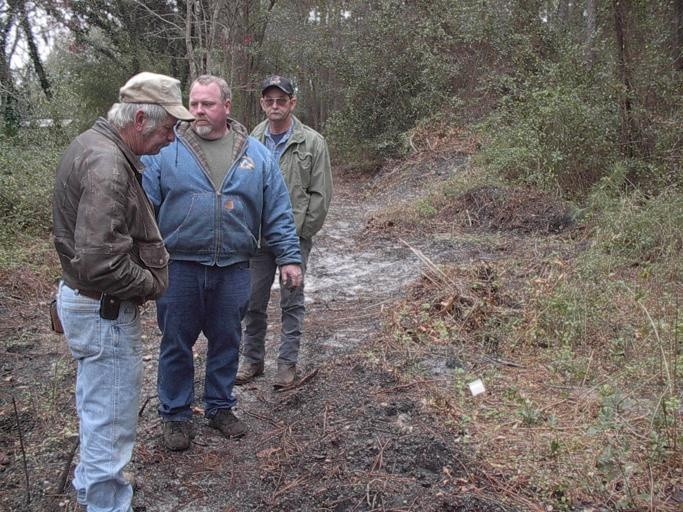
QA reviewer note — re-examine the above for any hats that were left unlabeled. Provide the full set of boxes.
[262,75,293,95]
[119,71,196,122]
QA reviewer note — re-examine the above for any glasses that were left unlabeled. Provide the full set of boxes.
[263,97,290,106]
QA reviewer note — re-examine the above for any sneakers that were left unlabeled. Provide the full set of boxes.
[160,422,191,451]
[235,359,264,384]
[204,407,248,438]
[272,363,296,388]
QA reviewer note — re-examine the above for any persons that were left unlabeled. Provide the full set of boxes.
[232,74,333,389]
[50,70,197,511]
[144,75,303,452]
